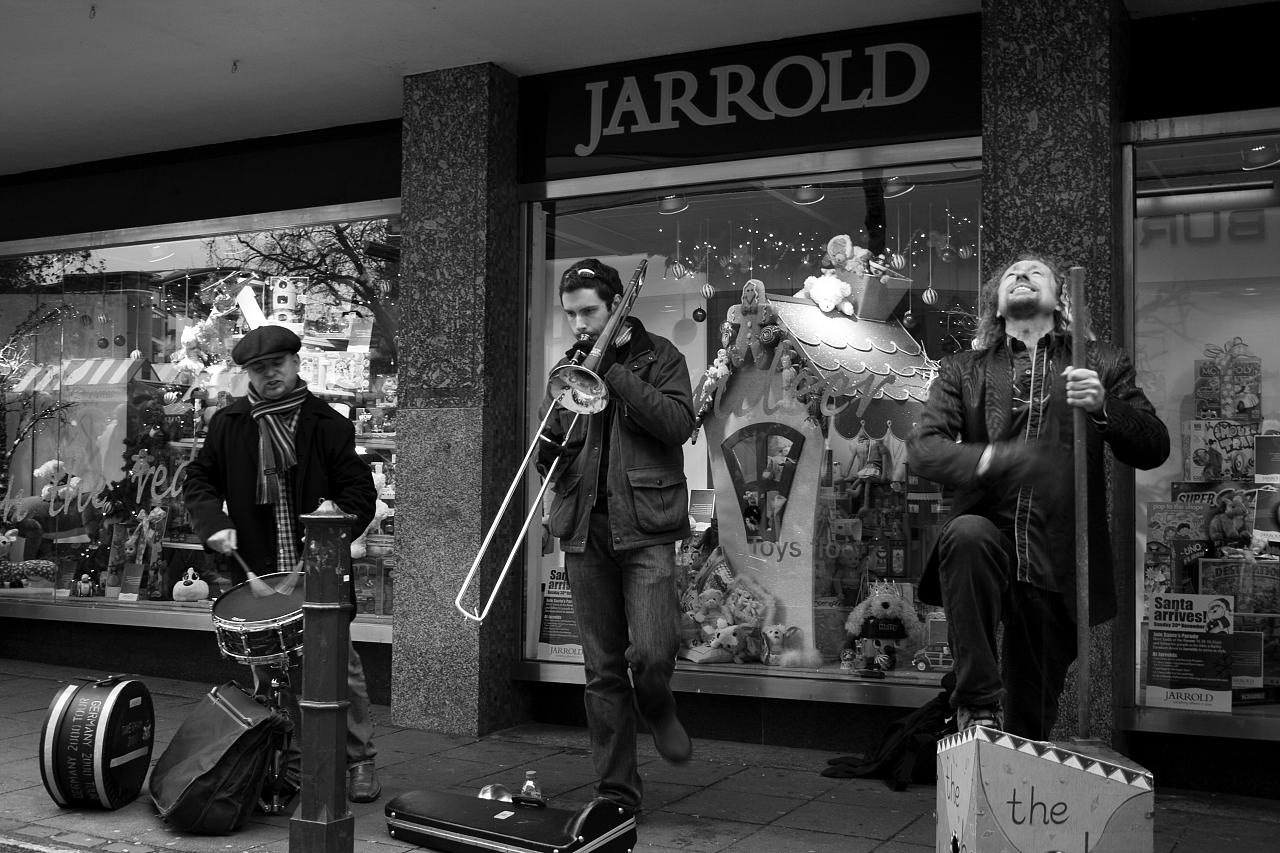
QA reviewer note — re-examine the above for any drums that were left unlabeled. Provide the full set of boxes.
[210,569,306,667]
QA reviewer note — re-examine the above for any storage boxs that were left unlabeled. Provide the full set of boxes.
[1171,540,1280,636]
[1183,358,1262,481]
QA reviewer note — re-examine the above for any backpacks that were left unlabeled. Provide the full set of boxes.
[149,681,295,835]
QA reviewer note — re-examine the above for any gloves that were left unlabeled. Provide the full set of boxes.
[563,339,616,376]
[540,430,569,466]
[987,441,1051,483]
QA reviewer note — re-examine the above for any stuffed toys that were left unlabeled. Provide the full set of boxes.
[682,590,825,668]
[844,585,922,668]
[34,460,81,503]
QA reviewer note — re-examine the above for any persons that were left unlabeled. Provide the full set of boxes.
[182,326,381,801]
[904,252,1171,743]
[532,258,713,805]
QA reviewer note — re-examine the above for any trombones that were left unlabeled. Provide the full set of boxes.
[453,257,652,624]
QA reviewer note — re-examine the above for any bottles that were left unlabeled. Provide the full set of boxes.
[521,771,542,805]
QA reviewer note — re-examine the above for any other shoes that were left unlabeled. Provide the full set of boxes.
[957,706,998,733]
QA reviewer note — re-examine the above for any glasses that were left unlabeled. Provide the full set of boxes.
[564,269,614,292]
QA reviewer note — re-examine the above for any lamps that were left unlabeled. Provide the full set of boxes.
[1240,139,1280,173]
[790,185,826,206]
[882,176,915,199]
[148,244,175,264]
[658,196,691,216]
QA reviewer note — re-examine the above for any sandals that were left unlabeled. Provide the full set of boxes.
[650,714,690,761]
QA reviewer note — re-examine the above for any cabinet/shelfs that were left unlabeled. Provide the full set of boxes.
[161,430,395,550]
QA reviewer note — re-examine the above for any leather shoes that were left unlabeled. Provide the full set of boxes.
[347,762,379,802]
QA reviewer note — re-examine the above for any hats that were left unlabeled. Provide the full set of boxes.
[231,325,301,369]
